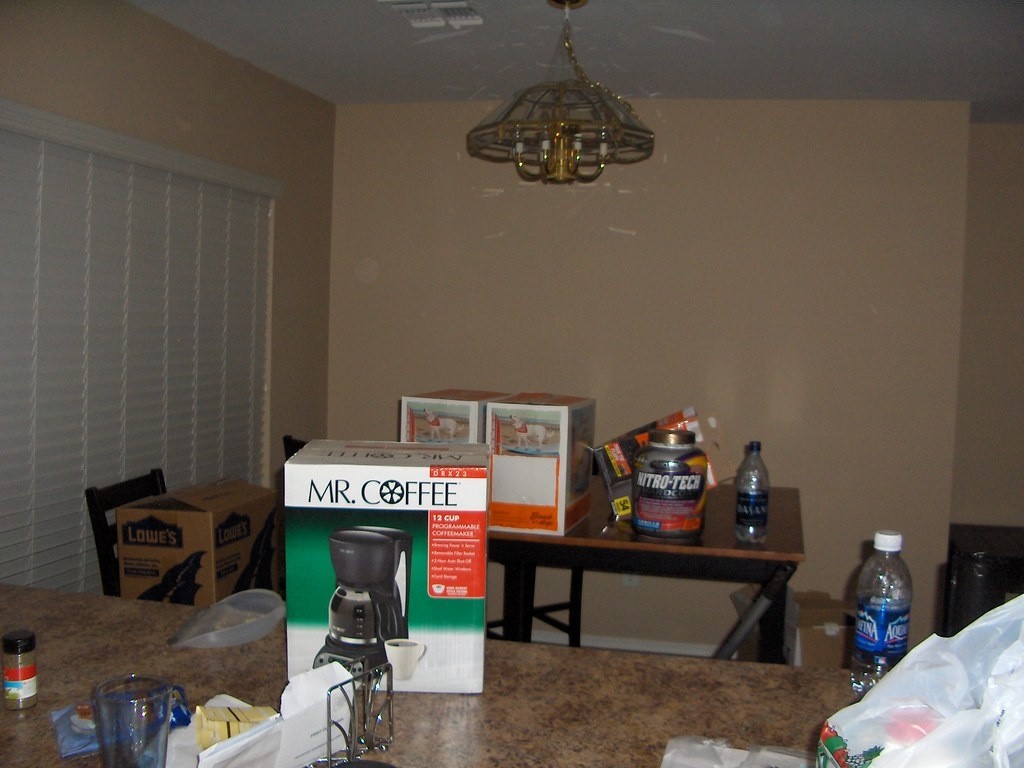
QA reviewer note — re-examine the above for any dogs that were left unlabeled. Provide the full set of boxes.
[510,415,555,452]
[424,408,465,443]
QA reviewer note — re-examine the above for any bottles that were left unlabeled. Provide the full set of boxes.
[630,428,708,544]
[850,530,913,693]
[1,630,39,710]
[734,442,769,546]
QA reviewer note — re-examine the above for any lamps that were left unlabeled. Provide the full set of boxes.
[466,0,655,184]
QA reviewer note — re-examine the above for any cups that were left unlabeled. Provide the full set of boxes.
[90,674,173,768]
[384,638,428,680]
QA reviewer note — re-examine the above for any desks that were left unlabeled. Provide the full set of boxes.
[0,581,863,768]
[946,523,1024,637]
[488,486,805,666]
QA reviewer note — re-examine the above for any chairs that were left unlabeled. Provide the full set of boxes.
[86,468,166,598]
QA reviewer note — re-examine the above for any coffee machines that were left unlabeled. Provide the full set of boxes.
[312,525,413,677]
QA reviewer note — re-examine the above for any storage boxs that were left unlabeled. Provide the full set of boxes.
[115,477,284,608]
[284,439,488,694]
[485,392,596,537]
[729,581,858,668]
[594,405,737,518]
[401,389,518,443]
